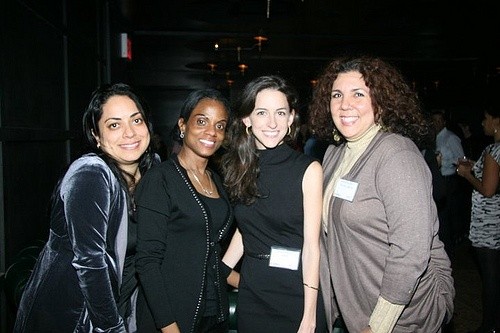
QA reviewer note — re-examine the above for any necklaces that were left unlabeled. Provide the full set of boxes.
[177,154,214,196]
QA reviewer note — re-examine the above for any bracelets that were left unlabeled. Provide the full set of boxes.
[304,284,319,291]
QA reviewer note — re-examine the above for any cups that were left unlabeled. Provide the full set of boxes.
[458,154,472,170]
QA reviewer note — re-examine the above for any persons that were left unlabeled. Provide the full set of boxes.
[13,83,160,333]
[133,87,241,333]
[305,110,479,246]
[215,75,323,333]
[456,104,500,333]
[309,54,456,333]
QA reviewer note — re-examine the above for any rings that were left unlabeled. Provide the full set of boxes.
[457,169,459,171]
